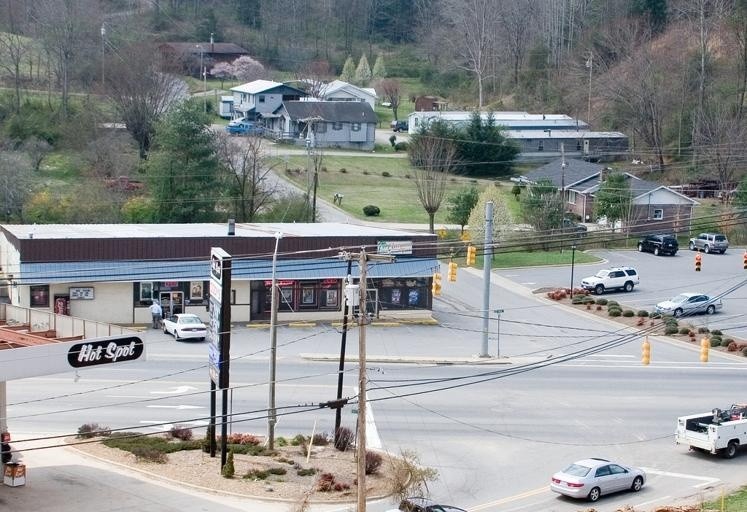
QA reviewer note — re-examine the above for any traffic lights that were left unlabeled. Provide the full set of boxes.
[447,262,457,281]
[695,254,701,271]
[743,253,746,269]
[467,246,477,265]
[432,273,441,296]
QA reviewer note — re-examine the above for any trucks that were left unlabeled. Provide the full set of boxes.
[688,179,738,198]
[676,403,747,458]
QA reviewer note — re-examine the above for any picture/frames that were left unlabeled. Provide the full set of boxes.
[190,282,204,300]
[230,290,235,305]
[140,282,153,301]
[69,287,94,300]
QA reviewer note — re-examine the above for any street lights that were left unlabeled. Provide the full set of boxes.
[266,231,284,451]
[304,137,312,224]
[585,51,594,132]
[99,23,105,95]
[569,241,578,298]
[559,161,567,255]
[195,45,203,80]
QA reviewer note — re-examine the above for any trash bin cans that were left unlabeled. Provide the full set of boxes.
[4,463,26,487]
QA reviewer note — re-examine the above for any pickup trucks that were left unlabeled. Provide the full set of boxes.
[225,123,262,137]
[105,176,144,192]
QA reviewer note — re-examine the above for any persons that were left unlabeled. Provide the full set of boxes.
[149,301,162,328]
[192,285,201,297]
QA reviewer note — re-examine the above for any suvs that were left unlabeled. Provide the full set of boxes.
[689,232,728,254]
[390,120,408,132]
[582,266,640,294]
[638,233,678,256]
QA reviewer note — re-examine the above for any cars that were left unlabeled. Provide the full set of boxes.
[162,312,207,342]
[550,457,648,501]
[387,497,467,511]
[228,117,256,128]
[655,293,723,318]
